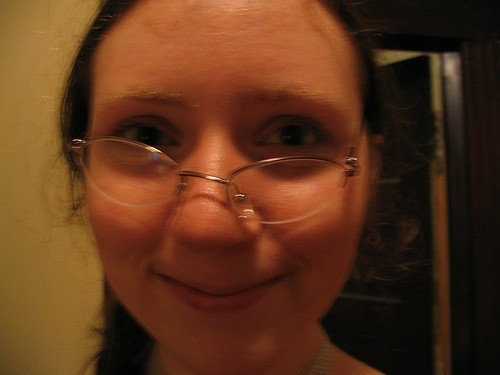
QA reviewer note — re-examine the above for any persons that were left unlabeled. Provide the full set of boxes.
[59,2,395,375]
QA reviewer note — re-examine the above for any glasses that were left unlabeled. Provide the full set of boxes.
[69,110,375,224]
